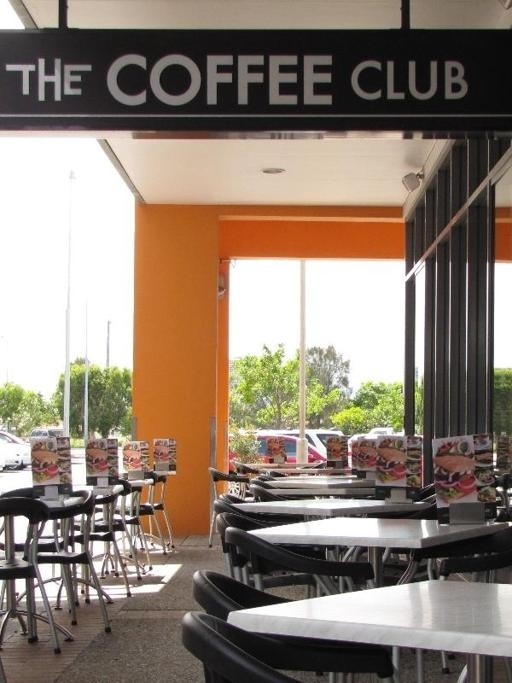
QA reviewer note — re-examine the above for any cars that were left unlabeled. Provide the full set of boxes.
[228,427,396,473]
[0,431,31,473]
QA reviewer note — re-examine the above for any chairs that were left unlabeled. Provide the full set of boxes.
[181,613,392,683]
[394,527,512,674]
[0,465,180,682]
[191,570,387,679]
[225,527,399,675]
[215,513,317,583]
[205,456,512,549]
